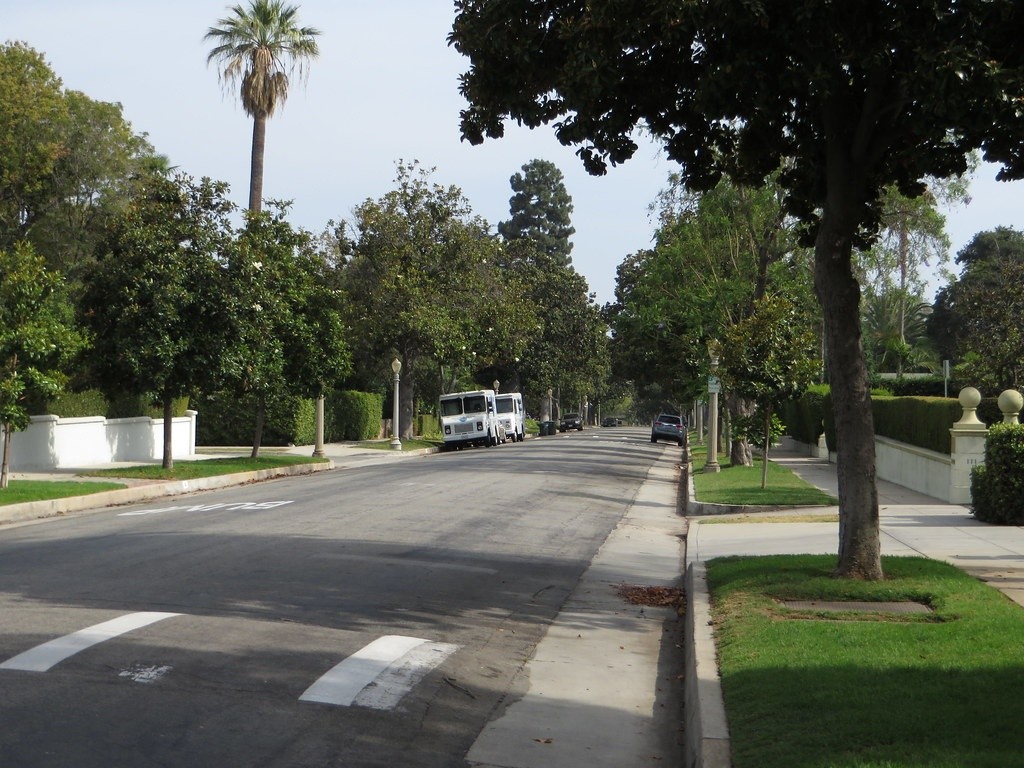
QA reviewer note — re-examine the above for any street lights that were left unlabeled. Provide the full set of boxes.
[388,357,403,451]
[582,395,587,425]
[598,400,601,426]
[493,379,500,395]
[703,336,722,472]
[547,388,553,422]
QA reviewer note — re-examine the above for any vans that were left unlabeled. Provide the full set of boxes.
[438,388,507,451]
[495,392,527,443]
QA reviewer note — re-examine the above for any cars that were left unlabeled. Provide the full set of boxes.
[651,412,689,447]
[558,412,583,432]
[601,417,616,427]
